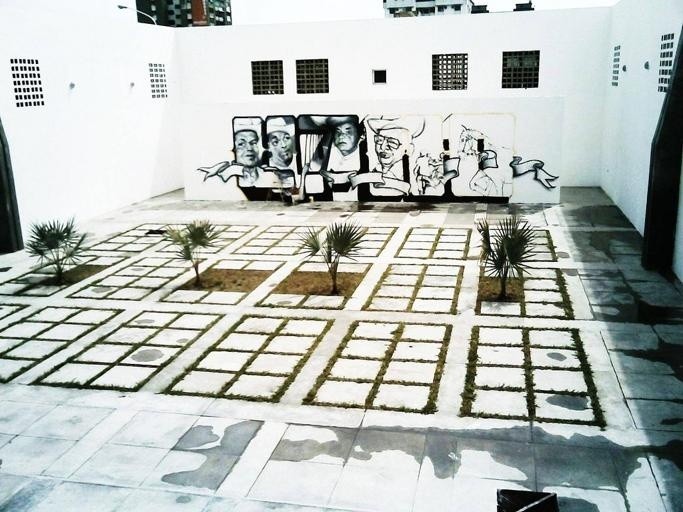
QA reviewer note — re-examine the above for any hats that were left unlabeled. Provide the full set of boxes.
[266,118,295,136]
[367,114,425,136]
[235,118,261,133]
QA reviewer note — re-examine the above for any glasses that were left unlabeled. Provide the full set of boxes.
[374,135,400,149]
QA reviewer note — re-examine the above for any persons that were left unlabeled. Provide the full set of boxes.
[234,129,265,187]
[268,131,301,188]
[372,123,414,181]
[326,122,360,172]
